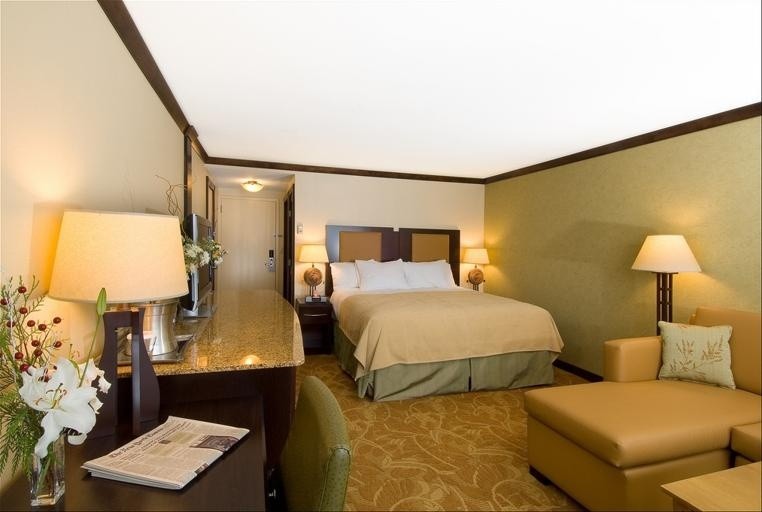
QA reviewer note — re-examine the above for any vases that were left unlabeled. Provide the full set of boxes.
[24,425,67,505]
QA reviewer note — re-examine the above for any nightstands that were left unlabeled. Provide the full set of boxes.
[296,299,332,355]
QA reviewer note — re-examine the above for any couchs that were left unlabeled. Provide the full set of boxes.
[524,306,762,512]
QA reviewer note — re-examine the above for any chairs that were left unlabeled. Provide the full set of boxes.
[267,376,351,512]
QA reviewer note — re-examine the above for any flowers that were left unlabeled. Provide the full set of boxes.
[0,274,108,478]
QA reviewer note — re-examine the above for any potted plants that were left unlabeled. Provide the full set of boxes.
[125,234,225,358]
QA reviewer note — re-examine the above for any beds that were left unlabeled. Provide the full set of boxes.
[326,260,564,401]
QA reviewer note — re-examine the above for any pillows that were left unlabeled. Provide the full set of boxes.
[356,259,406,290]
[331,259,375,291]
[398,259,456,289]
[657,319,737,392]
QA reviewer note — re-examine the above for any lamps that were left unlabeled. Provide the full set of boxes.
[629,234,702,336]
[240,181,263,192]
[462,249,490,291]
[46,213,190,440]
[299,244,329,302]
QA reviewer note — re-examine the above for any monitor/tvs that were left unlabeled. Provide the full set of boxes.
[178,213,215,319]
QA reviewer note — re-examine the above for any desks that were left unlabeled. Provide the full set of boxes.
[659,460,762,512]
[116,290,305,465]
[0,393,265,511]
